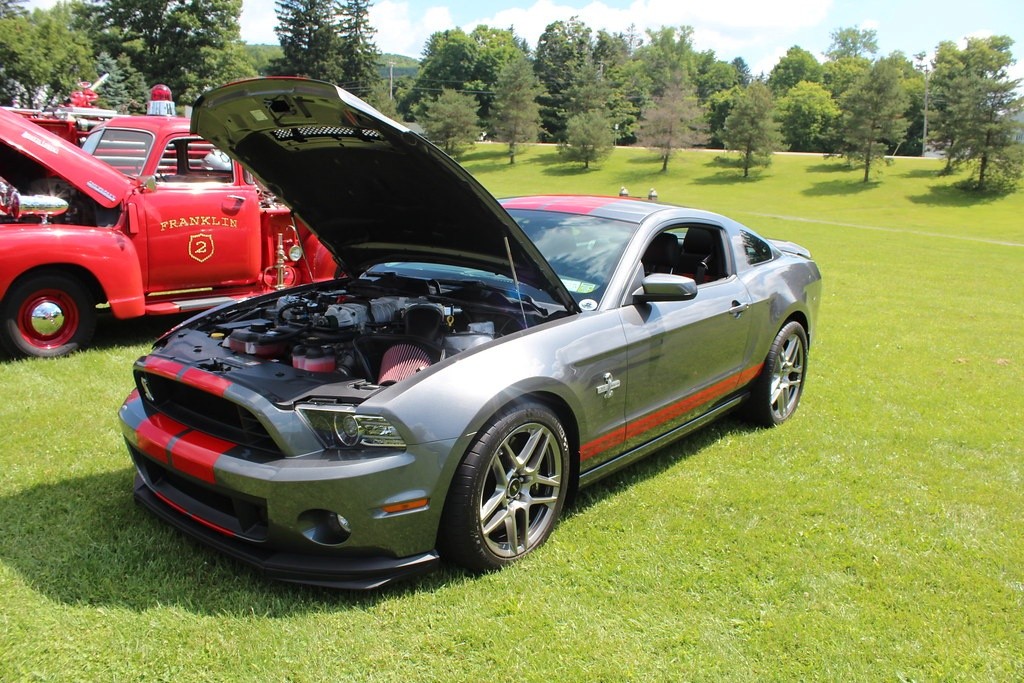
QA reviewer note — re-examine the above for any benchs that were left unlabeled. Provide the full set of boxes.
[158,175,231,184]
[675,228,721,282]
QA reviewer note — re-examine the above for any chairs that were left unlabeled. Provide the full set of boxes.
[541,230,577,254]
[643,232,679,275]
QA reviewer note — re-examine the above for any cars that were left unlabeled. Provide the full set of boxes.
[0,83,346,360]
[117,76,822,592]
